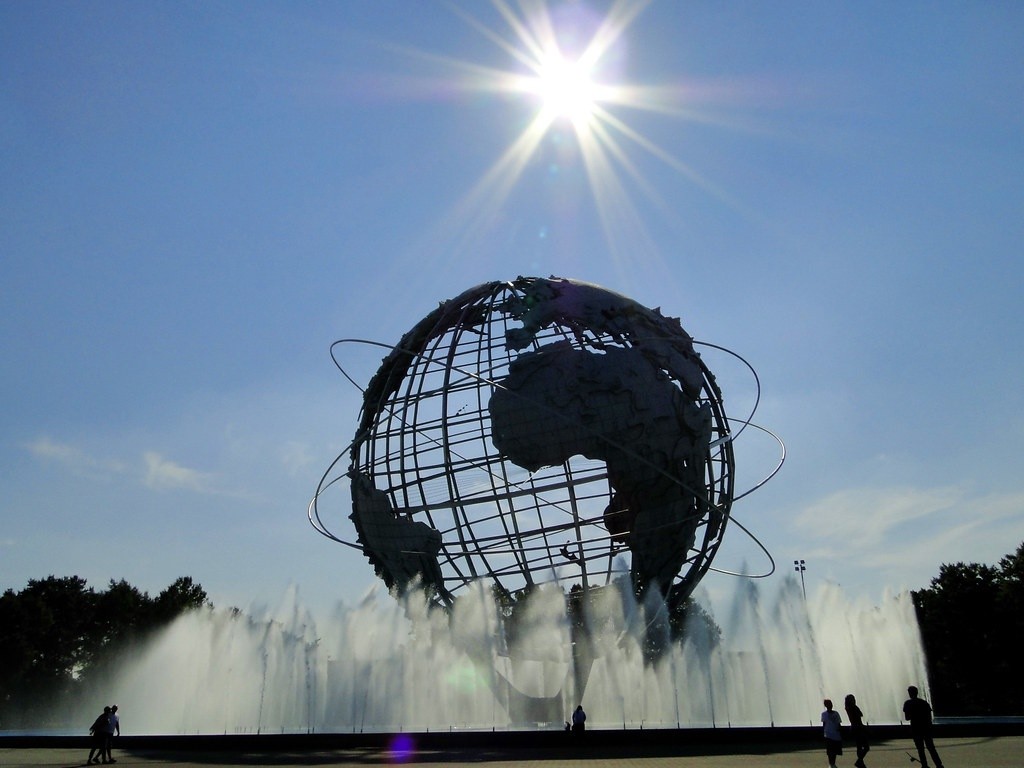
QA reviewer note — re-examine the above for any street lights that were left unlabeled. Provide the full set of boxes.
[794,559,807,601]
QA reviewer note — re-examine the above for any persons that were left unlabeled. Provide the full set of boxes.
[565,705,586,732]
[903,686,945,768]
[821,700,843,768]
[86,705,120,765]
[845,695,870,768]
